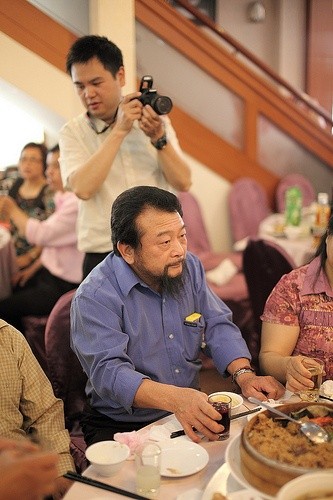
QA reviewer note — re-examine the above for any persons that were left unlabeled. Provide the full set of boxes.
[0,143,333,500]
[58,36,193,281]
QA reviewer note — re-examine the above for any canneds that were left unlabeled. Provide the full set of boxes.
[285,186,302,225]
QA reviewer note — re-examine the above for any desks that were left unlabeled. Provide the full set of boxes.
[258,233,317,267]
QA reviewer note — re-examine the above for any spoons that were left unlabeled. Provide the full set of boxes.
[247,396,328,443]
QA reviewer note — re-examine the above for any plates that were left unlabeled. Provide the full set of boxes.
[142,439,210,478]
[284,380,333,403]
[202,433,275,500]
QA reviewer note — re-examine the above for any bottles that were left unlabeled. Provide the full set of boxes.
[313,193,330,248]
[283,185,302,226]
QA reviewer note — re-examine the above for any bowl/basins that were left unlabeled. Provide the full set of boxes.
[208,392,243,415]
[276,471,333,500]
[85,441,130,477]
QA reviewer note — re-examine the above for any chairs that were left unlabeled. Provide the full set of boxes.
[179,172,317,358]
[44,288,91,474]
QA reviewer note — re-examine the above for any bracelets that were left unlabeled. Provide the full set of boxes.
[232,366,256,392]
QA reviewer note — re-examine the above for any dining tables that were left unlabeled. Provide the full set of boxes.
[63,388,333,500]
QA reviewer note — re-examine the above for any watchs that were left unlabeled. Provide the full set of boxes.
[151,131,168,150]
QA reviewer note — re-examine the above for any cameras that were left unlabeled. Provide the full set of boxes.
[135,76,172,116]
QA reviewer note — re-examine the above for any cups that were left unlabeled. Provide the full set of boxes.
[270,213,300,241]
[299,358,324,403]
[134,443,161,498]
[208,394,232,441]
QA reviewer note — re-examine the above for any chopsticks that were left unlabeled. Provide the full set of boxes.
[63,470,150,500]
[170,407,262,438]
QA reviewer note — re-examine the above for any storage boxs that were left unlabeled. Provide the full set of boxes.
[185,313,202,323]
